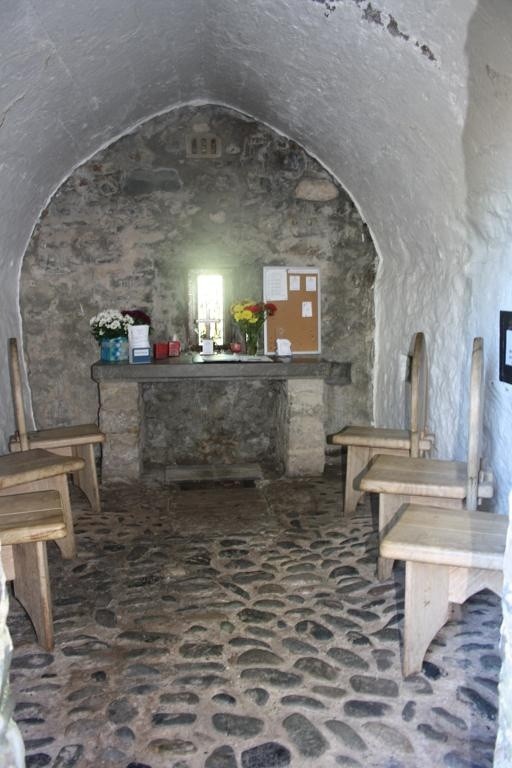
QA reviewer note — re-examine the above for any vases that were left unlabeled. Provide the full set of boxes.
[101,336,129,361]
[242,331,260,356]
[128,325,151,346]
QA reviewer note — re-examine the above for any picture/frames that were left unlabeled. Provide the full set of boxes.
[495,307,511,386]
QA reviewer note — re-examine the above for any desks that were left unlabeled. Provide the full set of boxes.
[87,353,334,505]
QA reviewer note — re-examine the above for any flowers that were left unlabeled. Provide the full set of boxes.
[229,295,276,351]
[121,308,154,336]
[89,308,134,345]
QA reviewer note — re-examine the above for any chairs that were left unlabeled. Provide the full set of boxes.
[353,330,498,584]
[6,336,110,516]
[326,329,435,518]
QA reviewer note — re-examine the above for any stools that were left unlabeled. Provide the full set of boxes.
[0,443,90,567]
[0,491,75,655]
[376,501,512,682]
